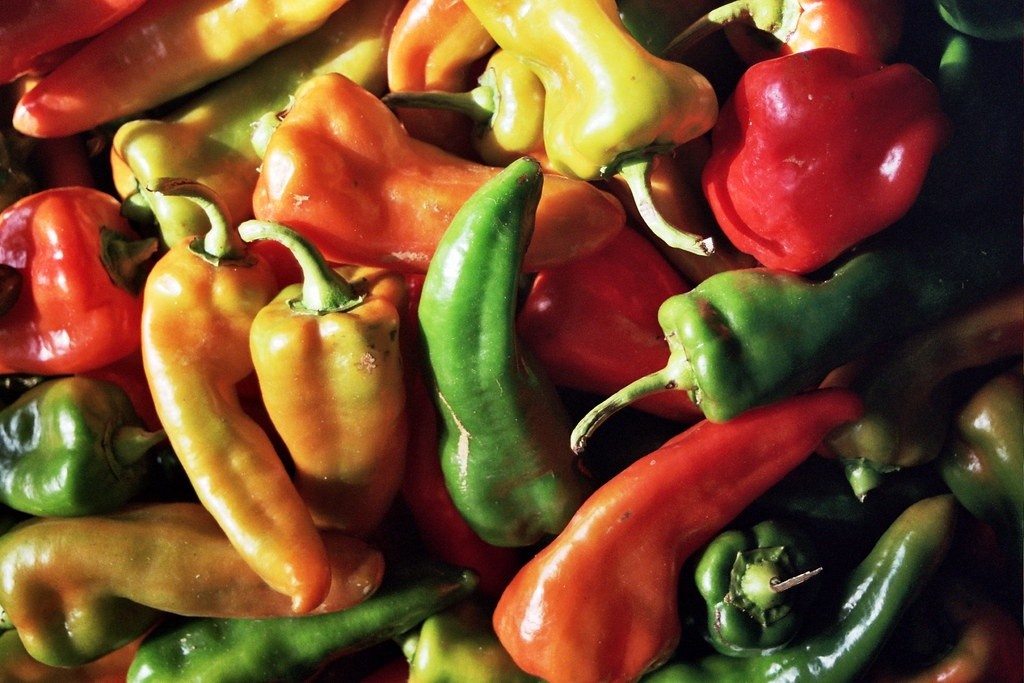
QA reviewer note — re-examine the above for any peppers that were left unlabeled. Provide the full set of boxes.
[0,1,1024,682]
